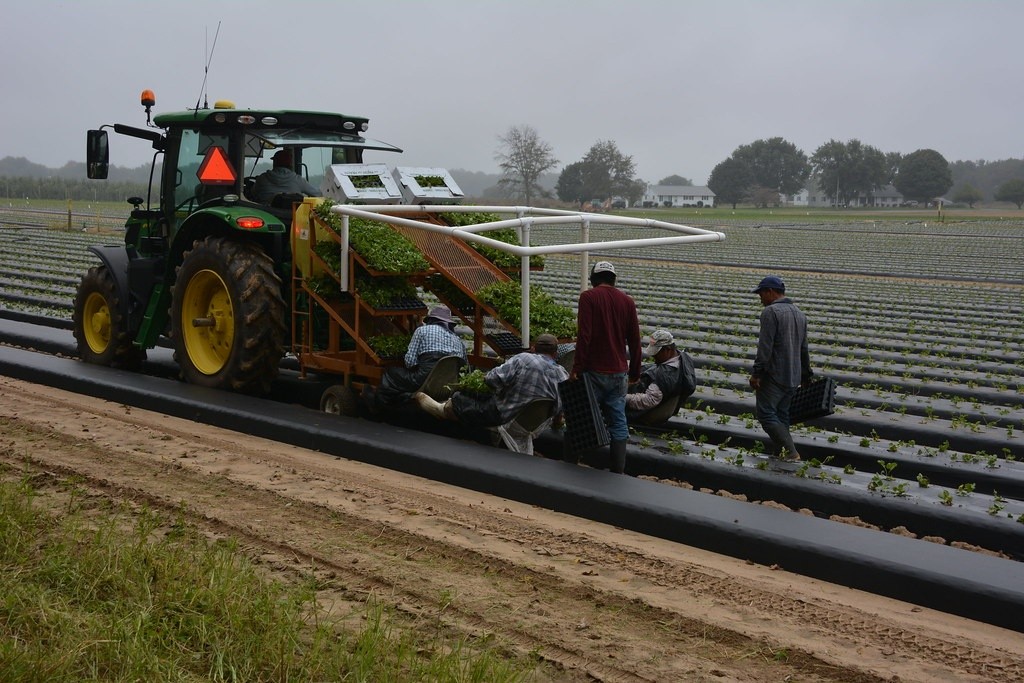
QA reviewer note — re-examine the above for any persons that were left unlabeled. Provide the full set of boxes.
[363,306,470,412]
[749,276,813,459]
[625,330,696,427]
[242,150,322,204]
[414,332,571,428]
[569,260,639,472]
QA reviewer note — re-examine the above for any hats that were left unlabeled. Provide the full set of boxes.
[751,277,786,294]
[423,307,458,330]
[536,333,558,346]
[644,330,676,356]
[590,261,618,277]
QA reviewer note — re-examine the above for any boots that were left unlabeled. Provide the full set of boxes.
[765,424,800,461]
[415,392,459,421]
[609,438,626,474]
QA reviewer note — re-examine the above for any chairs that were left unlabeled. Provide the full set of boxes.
[636,392,682,427]
[271,193,305,210]
[400,357,469,404]
[484,399,559,438]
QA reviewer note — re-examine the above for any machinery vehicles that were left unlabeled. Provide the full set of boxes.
[67,84,728,476]
